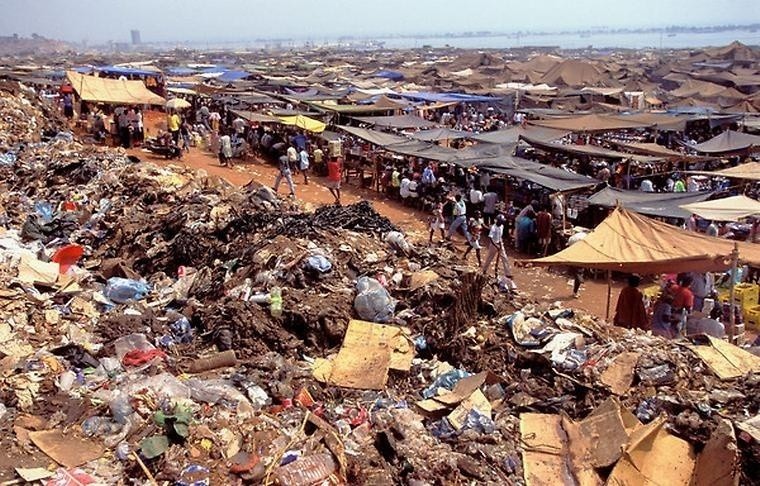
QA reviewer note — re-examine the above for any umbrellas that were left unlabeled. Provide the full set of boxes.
[166,98,192,108]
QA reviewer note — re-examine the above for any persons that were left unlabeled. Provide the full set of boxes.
[167,102,760,339]
[25,69,158,149]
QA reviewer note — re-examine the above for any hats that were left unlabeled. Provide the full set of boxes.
[438,177,445,183]
[497,214,507,221]
